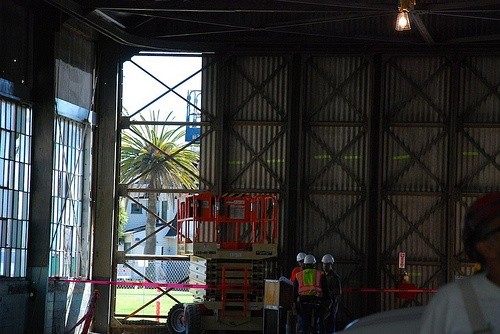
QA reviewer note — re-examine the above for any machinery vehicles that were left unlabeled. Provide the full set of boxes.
[167,194,279,333]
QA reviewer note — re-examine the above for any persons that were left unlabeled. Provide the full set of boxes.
[421,192,500,334]
[279,253,341,334]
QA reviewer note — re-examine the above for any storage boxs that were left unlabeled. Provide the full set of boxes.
[264,279,294,307]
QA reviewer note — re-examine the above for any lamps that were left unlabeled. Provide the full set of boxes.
[394,0,417,32]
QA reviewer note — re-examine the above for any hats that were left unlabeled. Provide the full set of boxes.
[296,252,307,262]
[304,255,317,265]
[463,193,496,248]
[320,254,335,265]
